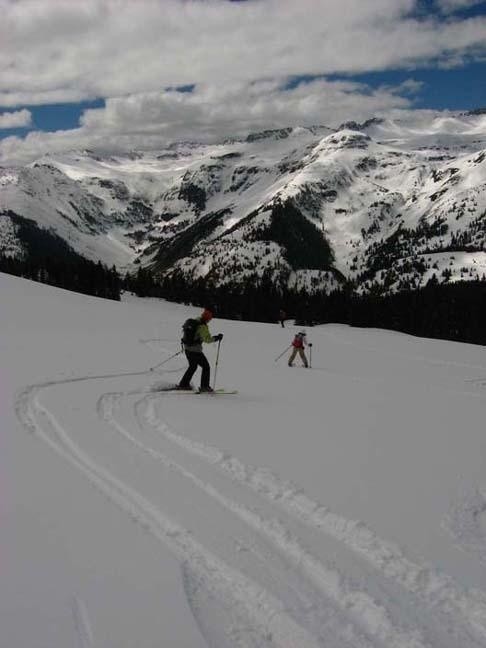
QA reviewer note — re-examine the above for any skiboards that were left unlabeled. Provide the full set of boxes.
[154,388,238,394]
[288,363,310,368]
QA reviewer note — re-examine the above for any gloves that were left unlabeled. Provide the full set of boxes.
[213,333,223,341]
[308,343,312,346]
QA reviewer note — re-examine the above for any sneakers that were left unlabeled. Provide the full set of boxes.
[179,384,215,392]
[288,363,308,368]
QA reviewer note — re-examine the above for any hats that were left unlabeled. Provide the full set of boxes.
[202,310,212,320]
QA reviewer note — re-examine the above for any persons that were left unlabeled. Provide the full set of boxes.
[285,331,314,368]
[181,309,223,393]
[279,309,286,329]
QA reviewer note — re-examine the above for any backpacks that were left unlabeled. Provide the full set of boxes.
[291,334,303,348]
[182,318,206,338]
